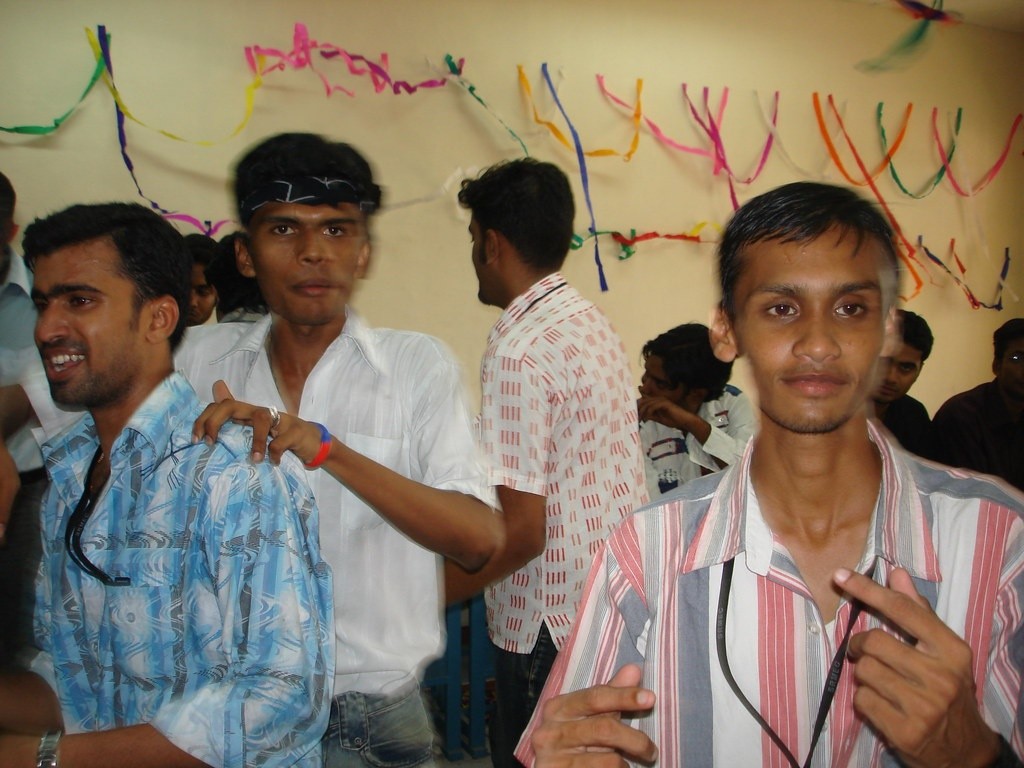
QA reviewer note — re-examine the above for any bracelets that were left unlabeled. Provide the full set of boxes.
[303,421,332,470]
[36,728,63,768]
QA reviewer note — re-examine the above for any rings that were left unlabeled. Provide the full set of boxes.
[268,407,281,428]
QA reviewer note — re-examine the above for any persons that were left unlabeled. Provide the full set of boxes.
[637,310,1023,490]
[437,156,652,768]
[0,133,508,768]
[511,182,1024,768]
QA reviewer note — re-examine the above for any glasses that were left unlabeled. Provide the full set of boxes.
[1003,350,1024,363]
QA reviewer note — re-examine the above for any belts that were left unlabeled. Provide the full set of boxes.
[19,466,47,486]
